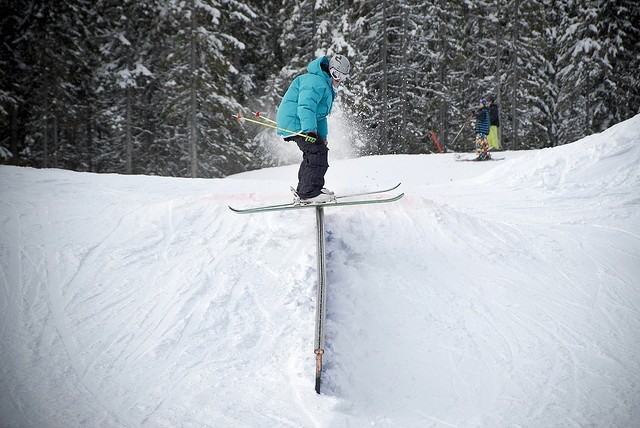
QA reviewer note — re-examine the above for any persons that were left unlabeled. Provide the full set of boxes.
[275,53,350,206]
[487,96,500,151]
[471,98,491,161]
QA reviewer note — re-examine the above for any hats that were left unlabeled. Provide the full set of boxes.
[479,97,486,104]
[328,54,350,74]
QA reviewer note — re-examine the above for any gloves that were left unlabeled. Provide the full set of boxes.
[323,139,328,146]
[305,132,317,143]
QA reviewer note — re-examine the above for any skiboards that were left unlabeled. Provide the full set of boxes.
[229,183,404,214]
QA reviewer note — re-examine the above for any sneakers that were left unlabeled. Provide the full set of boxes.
[321,186,334,194]
[300,192,333,202]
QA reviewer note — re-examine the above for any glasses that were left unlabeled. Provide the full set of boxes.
[330,68,347,81]
